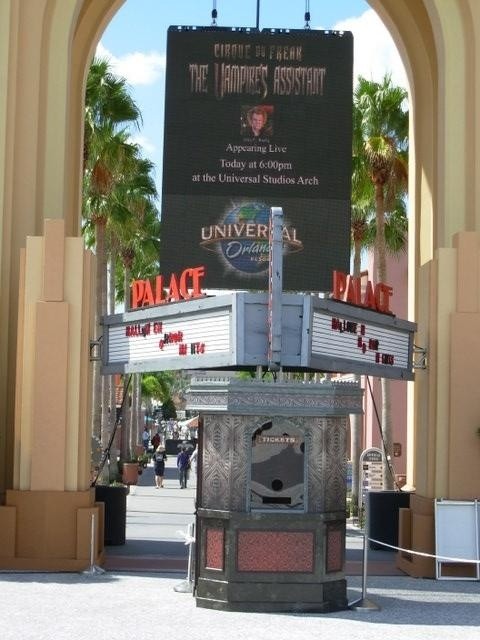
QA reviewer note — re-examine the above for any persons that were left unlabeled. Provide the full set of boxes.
[240,106,272,139]
[188,443,198,489]
[151,431,161,452]
[176,446,190,490]
[143,426,149,455]
[152,446,167,490]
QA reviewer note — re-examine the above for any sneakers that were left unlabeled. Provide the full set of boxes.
[156,485,186,489]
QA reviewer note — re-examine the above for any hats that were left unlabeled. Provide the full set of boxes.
[156,445,166,452]
